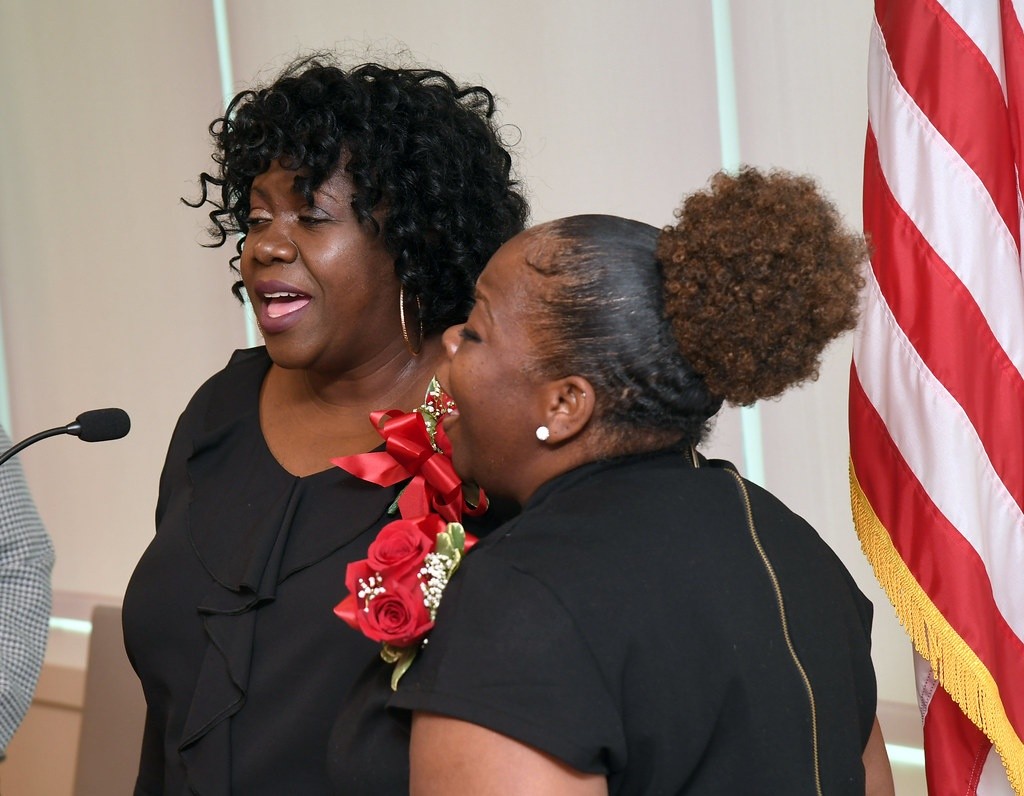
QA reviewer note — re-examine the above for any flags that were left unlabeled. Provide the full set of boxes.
[853,0,1024,796]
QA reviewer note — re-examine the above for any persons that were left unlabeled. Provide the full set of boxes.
[0,424,54,763]
[388,164,899,796]
[121,52,524,796]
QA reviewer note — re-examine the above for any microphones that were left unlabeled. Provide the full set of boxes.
[0,408,131,466]
